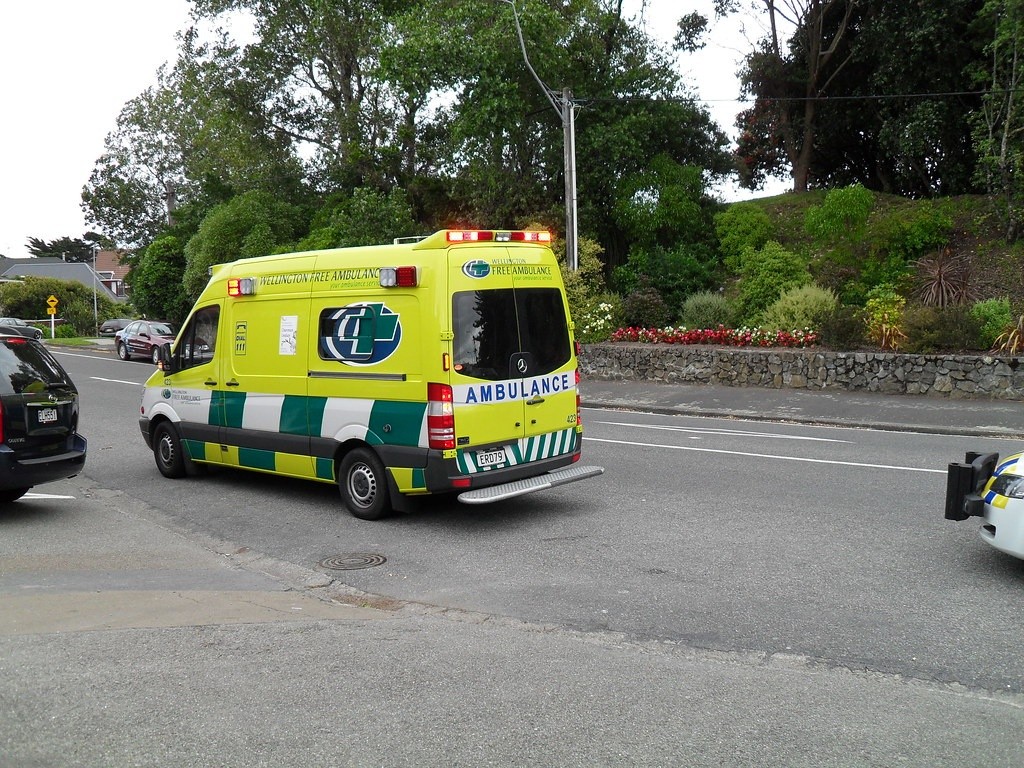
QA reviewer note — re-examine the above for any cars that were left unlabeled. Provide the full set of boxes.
[945,447,1024,562]
[0,325,88,502]
[0,317,43,340]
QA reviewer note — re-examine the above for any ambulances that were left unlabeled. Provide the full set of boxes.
[137,227,606,519]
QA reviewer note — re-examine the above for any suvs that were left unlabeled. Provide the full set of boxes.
[113,318,211,365]
[99,317,136,337]
